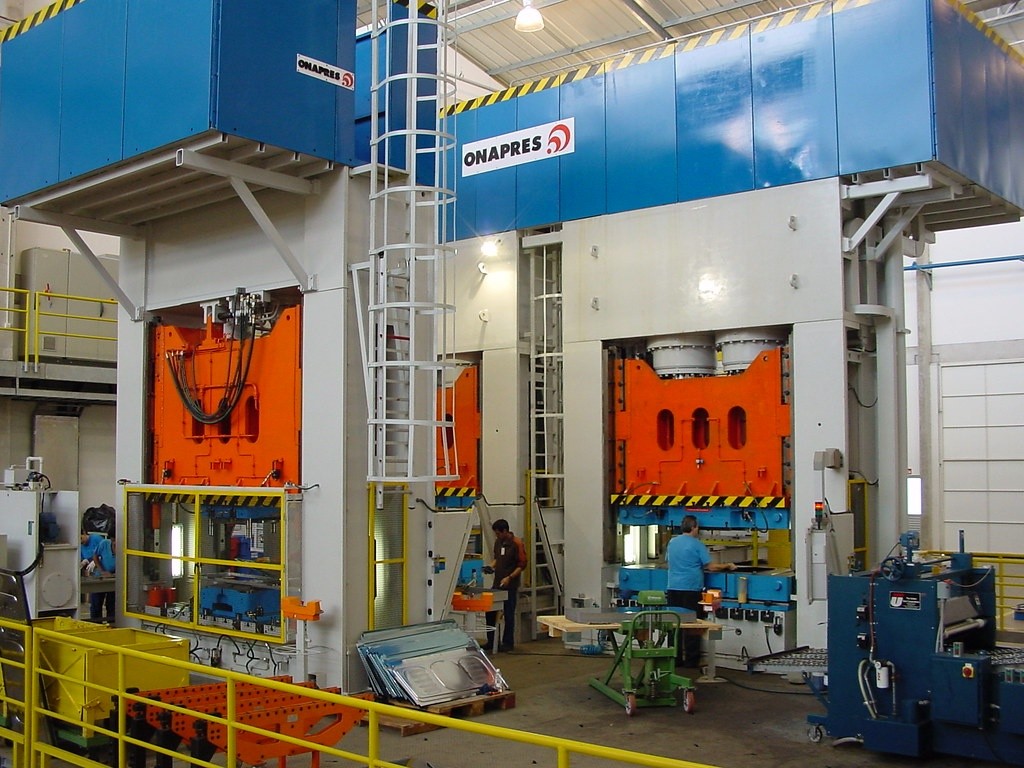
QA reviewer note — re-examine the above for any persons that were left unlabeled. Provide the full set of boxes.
[108,527,115,541]
[89,538,116,622]
[80,526,106,577]
[480,518,528,652]
[664,515,738,670]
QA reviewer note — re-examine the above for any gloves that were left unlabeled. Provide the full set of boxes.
[81,559,89,565]
[86,561,95,572]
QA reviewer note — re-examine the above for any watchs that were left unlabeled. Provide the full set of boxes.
[508,575,513,579]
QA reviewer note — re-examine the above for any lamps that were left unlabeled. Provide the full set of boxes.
[514,1,545,33]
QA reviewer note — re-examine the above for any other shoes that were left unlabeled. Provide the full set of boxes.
[503,644,514,651]
[480,642,493,650]
